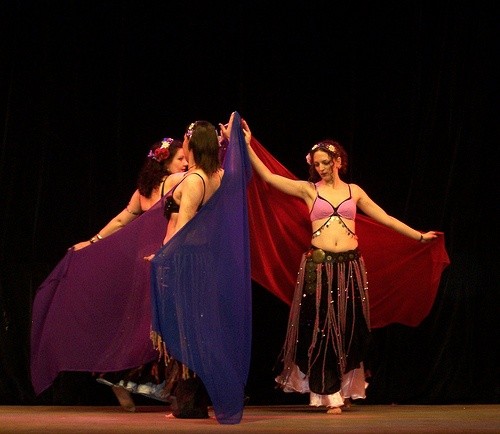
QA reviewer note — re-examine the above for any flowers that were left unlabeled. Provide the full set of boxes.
[186,122,194,141]
[305,144,336,166]
[148,137,173,162]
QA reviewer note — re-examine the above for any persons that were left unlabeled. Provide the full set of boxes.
[67,135,189,412]
[240,119,438,413]
[144,111,241,420]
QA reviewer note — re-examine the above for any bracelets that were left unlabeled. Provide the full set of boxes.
[418,233,424,242]
[94,233,103,241]
[89,238,97,243]
